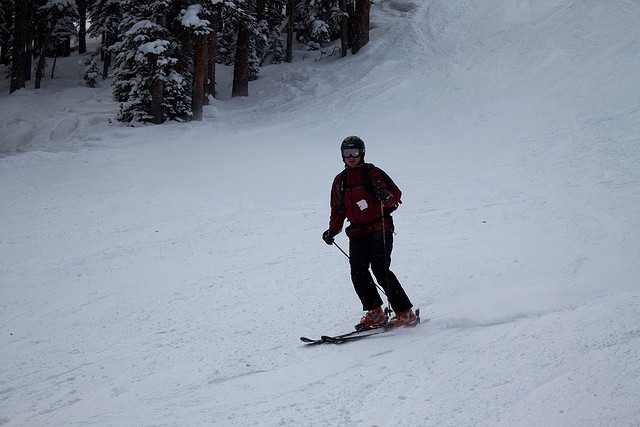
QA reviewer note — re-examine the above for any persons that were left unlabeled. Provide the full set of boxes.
[322,136,416,332]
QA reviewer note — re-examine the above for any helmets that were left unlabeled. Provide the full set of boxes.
[341,135,365,161]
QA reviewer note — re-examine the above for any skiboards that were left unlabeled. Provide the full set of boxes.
[301,318,419,345]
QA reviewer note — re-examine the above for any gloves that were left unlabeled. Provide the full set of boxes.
[322,229,338,244]
[377,187,390,199]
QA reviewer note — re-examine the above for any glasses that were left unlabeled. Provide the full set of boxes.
[341,148,361,158]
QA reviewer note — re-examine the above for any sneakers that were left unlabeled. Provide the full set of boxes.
[384,308,417,332]
[355,306,388,332]
